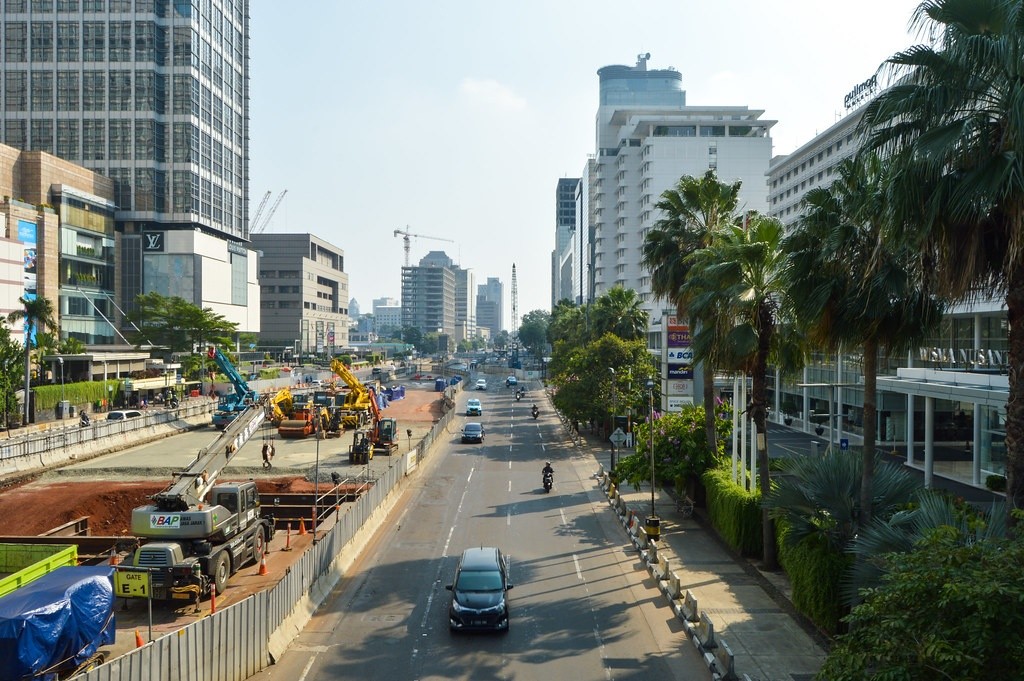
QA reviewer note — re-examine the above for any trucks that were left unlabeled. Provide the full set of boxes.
[0,540,80,598]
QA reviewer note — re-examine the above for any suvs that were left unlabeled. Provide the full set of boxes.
[466,398,482,416]
[445,546,513,638]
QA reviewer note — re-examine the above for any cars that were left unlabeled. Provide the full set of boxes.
[471,360,478,365]
[507,376,518,386]
[461,422,485,444]
[106,410,142,425]
[475,379,487,391]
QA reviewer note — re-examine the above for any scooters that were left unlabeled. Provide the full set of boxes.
[533,408,539,420]
[82,415,90,427]
[516,390,525,402]
[542,472,553,493]
[506,382,510,388]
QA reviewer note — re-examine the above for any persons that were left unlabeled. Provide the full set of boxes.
[542,461,554,483]
[81,412,90,425]
[516,389,520,398]
[531,404,539,415]
[164,388,176,403]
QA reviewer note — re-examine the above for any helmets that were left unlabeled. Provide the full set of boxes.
[83,412,85,414]
[546,462,550,466]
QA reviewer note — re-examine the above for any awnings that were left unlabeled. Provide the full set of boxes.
[119,375,200,391]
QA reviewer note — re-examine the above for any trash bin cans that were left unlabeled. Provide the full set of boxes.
[58,401,70,419]
[811,441,821,457]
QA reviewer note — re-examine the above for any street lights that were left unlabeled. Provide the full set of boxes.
[647,379,658,519]
[607,366,618,472]
[57,356,65,401]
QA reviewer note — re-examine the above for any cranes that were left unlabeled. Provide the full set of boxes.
[510,263,518,339]
[393,224,455,267]
[249,188,289,234]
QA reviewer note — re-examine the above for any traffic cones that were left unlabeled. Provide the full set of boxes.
[134,629,144,648]
[297,517,309,535]
[256,550,269,575]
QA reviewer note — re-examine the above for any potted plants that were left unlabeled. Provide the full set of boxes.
[766,395,774,418]
[810,409,829,436]
[779,402,797,426]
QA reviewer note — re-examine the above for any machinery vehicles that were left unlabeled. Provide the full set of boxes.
[205,344,376,440]
[116,392,277,610]
[349,424,373,465]
[367,388,399,457]
